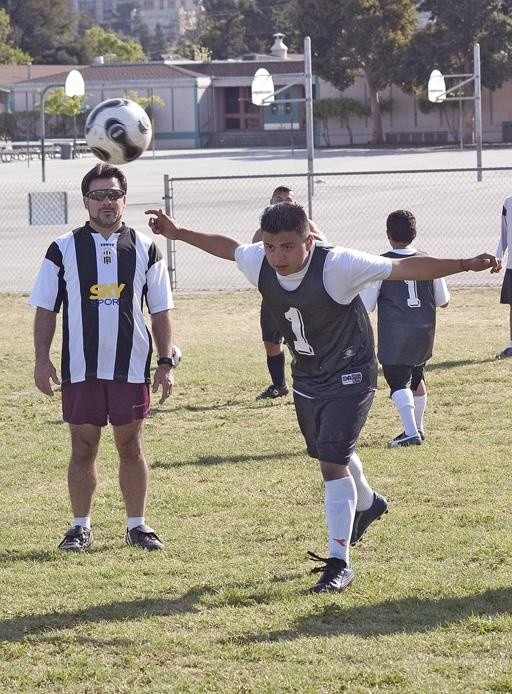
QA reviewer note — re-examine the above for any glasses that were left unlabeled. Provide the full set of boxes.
[84,189,126,200]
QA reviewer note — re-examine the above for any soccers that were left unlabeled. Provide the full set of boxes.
[157,345,182,368]
[84,98,152,164]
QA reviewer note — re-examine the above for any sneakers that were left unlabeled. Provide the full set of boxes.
[348,491,390,548]
[124,524,167,552]
[253,383,291,402]
[308,561,358,596]
[387,428,426,449]
[56,526,96,555]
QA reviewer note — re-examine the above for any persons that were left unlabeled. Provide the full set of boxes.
[358,208,450,447]
[246,185,331,401]
[27,164,180,551]
[142,202,504,597]
[489,196,512,359]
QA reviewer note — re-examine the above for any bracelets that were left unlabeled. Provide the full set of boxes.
[459,259,468,272]
[156,358,177,369]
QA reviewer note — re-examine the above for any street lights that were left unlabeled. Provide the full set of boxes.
[426,43,483,181]
[250,35,316,218]
[41,65,86,182]
